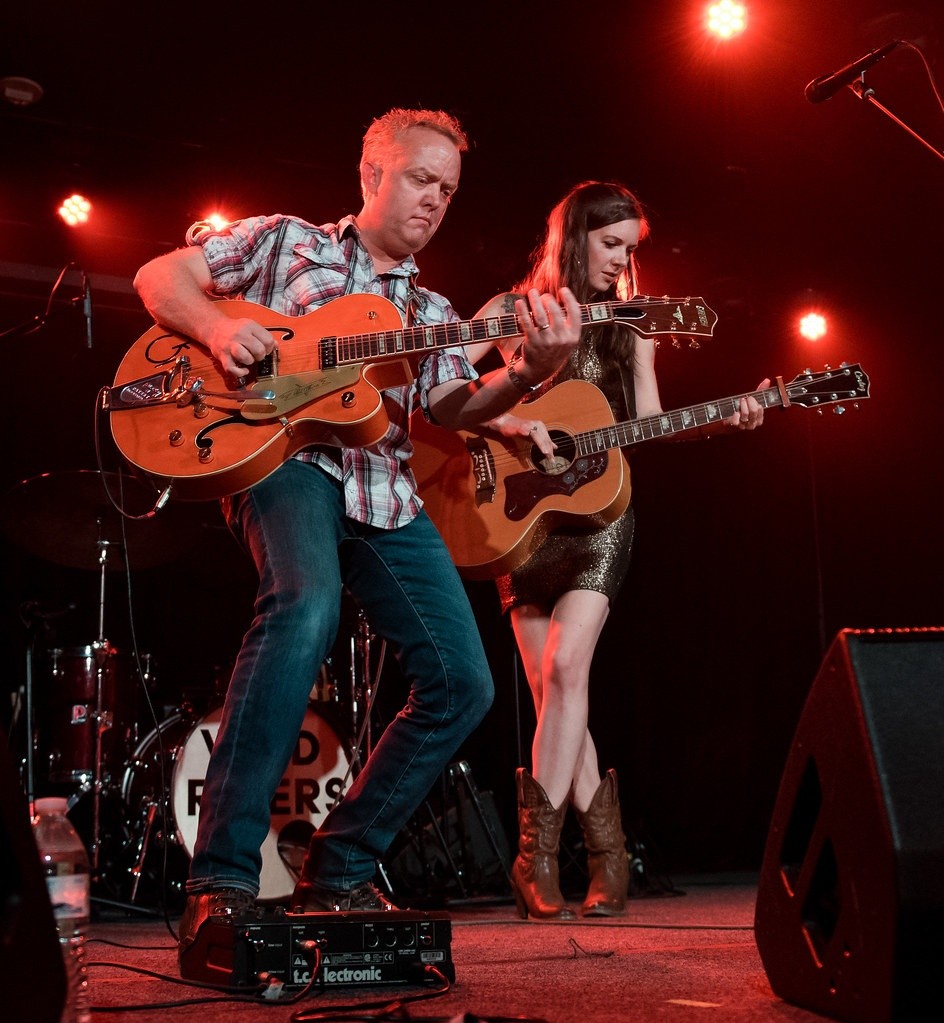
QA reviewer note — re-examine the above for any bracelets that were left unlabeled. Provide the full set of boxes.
[508,357,543,393]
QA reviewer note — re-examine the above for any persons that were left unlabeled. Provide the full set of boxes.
[464,181,772,918]
[133,106,580,990]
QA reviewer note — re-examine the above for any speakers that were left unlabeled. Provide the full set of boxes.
[387,790,513,900]
[0,764,68,1023]
[751,625,944,1023]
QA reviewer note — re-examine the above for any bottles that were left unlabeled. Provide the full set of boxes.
[25,797,93,1023]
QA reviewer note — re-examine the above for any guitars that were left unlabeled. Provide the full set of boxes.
[107,291,720,504]
[410,362,873,582]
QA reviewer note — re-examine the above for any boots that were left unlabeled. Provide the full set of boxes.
[511,768,575,921]
[571,769,629,918]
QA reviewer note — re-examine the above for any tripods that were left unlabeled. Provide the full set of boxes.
[62,517,123,885]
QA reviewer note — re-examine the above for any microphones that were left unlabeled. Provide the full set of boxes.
[82,275,93,348]
[803,41,900,105]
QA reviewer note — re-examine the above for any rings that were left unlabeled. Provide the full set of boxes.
[740,418,750,425]
[538,323,550,330]
[529,426,537,436]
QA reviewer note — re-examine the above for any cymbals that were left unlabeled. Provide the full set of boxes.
[2,467,214,574]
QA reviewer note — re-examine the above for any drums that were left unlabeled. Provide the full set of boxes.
[122,698,357,911]
[23,640,157,788]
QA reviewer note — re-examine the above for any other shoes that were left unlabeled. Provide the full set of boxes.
[290,873,401,912]
[178,889,254,957]
